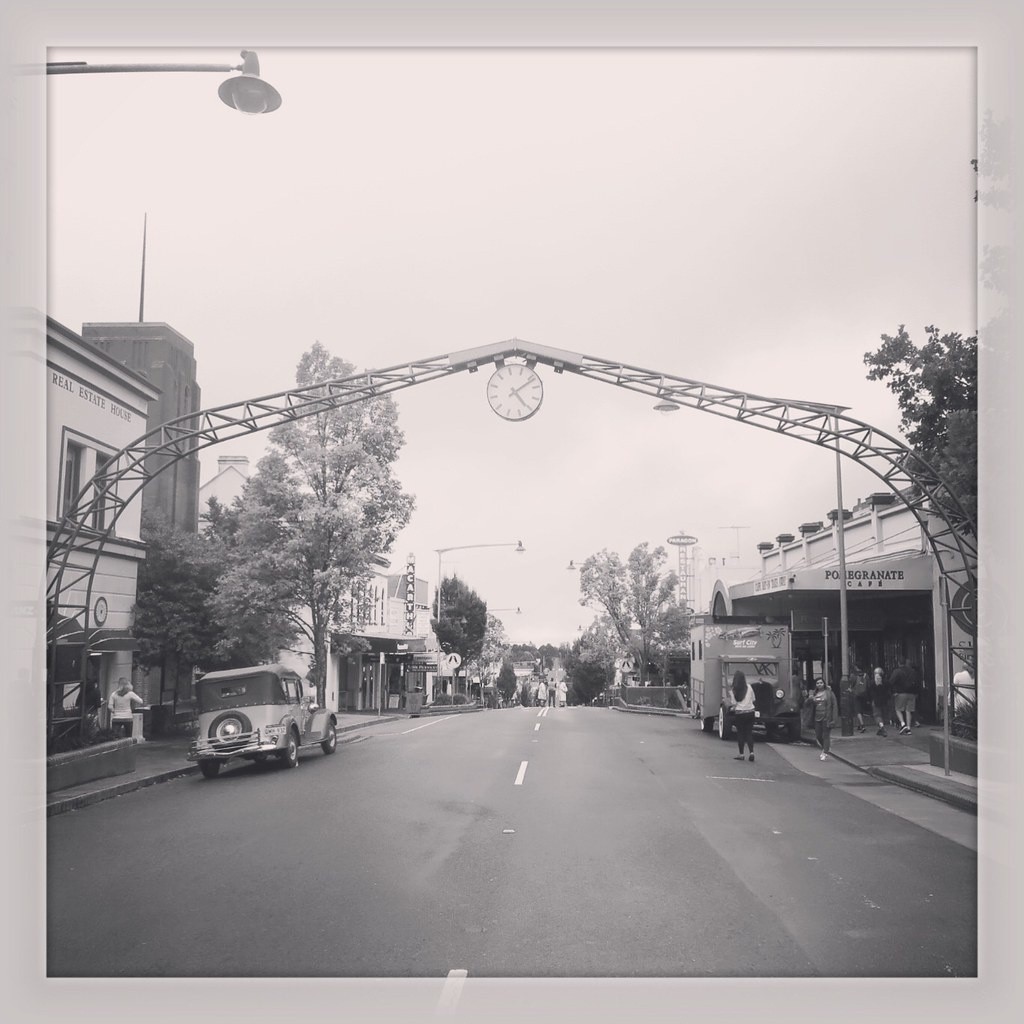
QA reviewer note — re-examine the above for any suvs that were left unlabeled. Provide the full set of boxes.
[187,663,337,777]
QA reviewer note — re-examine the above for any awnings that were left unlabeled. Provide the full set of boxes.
[86,627,141,652]
[47,611,86,645]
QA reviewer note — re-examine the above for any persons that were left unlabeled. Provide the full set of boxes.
[75,677,102,744]
[727,670,755,761]
[948,660,975,717]
[108,677,143,738]
[792,655,918,761]
[512,677,569,708]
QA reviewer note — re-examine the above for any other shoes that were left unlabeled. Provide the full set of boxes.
[881,726,887,737]
[906,730,911,734]
[734,756,744,760]
[820,750,827,761]
[749,755,754,761]
[899,725,907,733]
[860,728,866,733]
[877,730,882,735]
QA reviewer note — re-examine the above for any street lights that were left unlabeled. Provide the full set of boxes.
[464,608,522,696]
[436,539,526,697]
[653,390,854,738]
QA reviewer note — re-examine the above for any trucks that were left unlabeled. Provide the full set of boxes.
[690,624,802,740]
